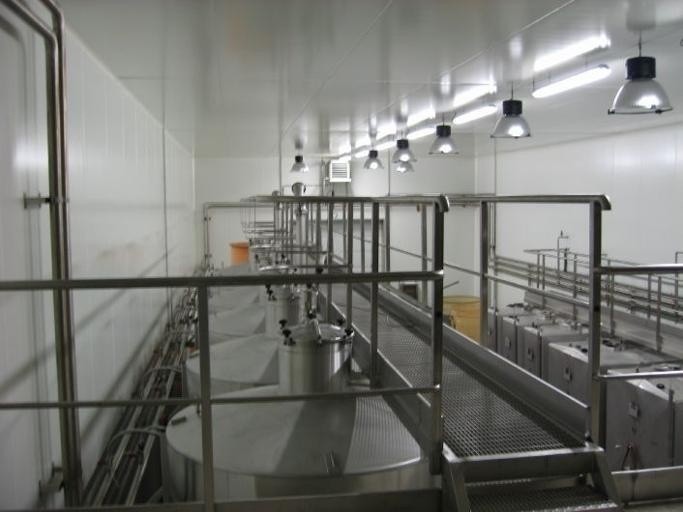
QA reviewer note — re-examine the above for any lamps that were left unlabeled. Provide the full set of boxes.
[363,148,384,172]
[487,82,534,142]
[426,113,460,157]
[451,100,497,126]
[405,124,436,142]
[606,34,674,119]
[327,132,397,161]
[530,63,612,100]
[291,154,309,172]
[391,137,417,174]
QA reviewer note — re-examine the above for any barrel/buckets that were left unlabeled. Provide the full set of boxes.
[229,241,247,265]
[444,295,481,340]
